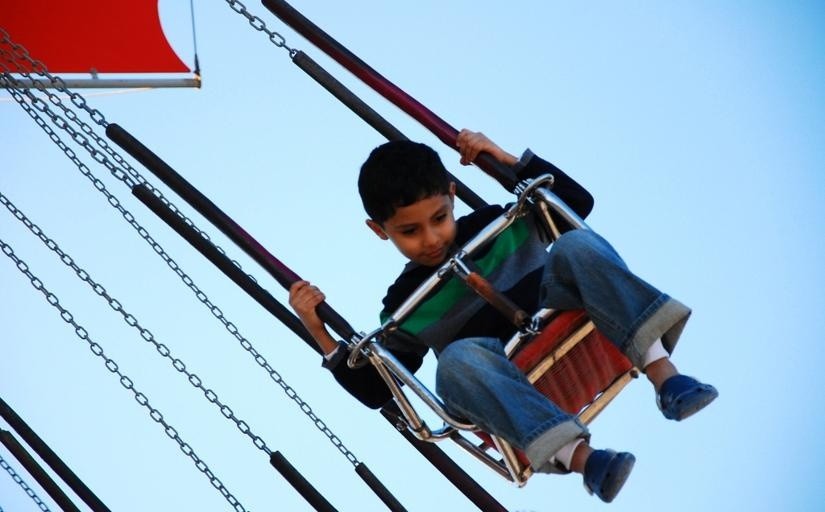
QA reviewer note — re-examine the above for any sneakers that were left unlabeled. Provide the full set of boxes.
[583,448,637,503]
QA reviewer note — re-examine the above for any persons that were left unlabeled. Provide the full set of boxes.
[287,125,720,503]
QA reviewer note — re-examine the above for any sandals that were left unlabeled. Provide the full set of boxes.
[656,375,720,422]
